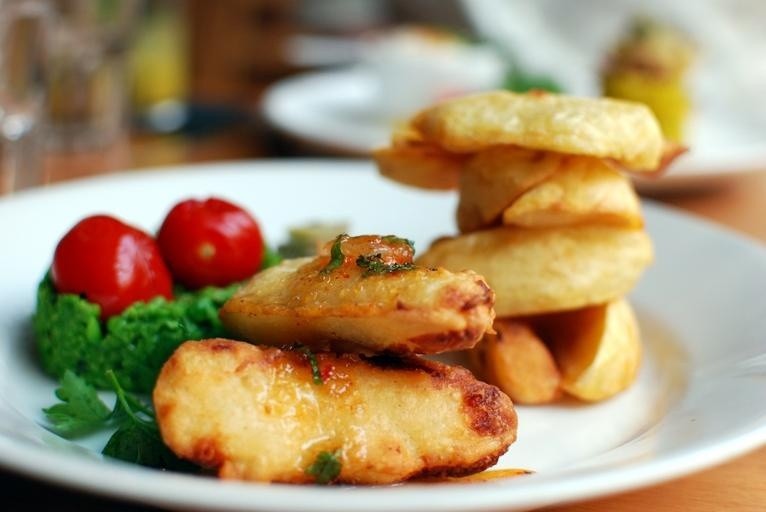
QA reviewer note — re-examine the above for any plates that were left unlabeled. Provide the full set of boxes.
[258,64,765,199]
[0,156,766,511]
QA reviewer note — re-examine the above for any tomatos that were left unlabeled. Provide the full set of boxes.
[157,196,262,290]
[52,216,172,321]
